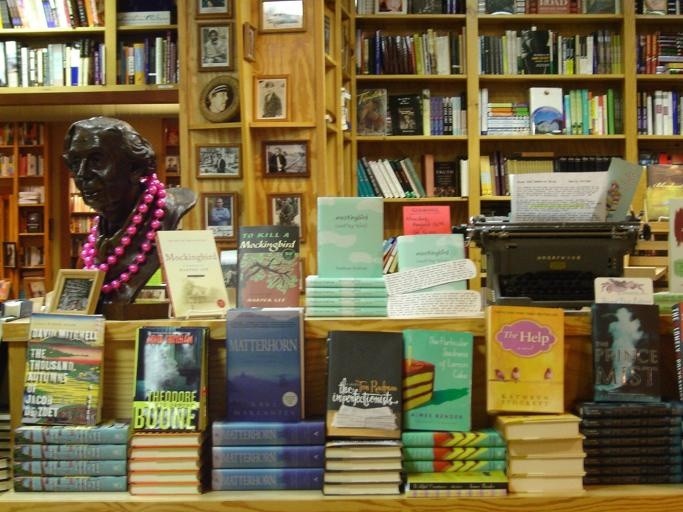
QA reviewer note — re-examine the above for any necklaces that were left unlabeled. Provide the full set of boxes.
[78,174,167,295]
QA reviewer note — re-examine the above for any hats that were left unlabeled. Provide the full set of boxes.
[209,84,228,97]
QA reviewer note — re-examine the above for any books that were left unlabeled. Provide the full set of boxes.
[402,205,451,236]
[528,87,565,135]
[357,0,375,15]
[305,274,388,318]
[646,164,683,221]
[18,120,45,146]
[459,159,469,197]
[0,122,14,145]
[235,225,300,307]
[479,30,523,75]
[17,191,41,204]
[0,154,13,177]
[0,413,13,493]
[316,197,384,278]
[636,89,683,136]
[356,26,468,76]
[667,198,683,295]
[412,0,443,15]
[400,428,509,499]
[375,0,408,16]
[479,87,530,136]
[422,88,468,136]
[434,161,459,197]
[670,302,683,404]
[211,418,326,492]
[355,88,388,136]
[69,215,100,232]
[520,30,553,75]
[155,229,231,320]
[325,329,405,441]
[0,0,106,29]
[131,326,211,433]
[383,237,397,274]
[494,414,587,494]
[126,431,210,496]
[563,87,623,136]
[402,328,473,432]
[389,94,424,136]
[18,152,44,177]
[408,0,412,15]
[13,419,131,493]
[593,276,654,306]
[573,399,683,486]
[636,0,683,15]
[0,38,106,87]
[591,303,662,404]
[68,177,82,194]
[224,307,305,423]
[116,30,178,86]
[19,246,43,266]
[478,0,623,15]
[485,305,565,415]
[116,11,171,25]
[553,28,625,75]
[356,154,435,199]
[637,30,683,75]
[69,195,97,211]
[443,0,467,15]
[638,149,683,167]
[20,312,106,427]
[397,234,467,292]
[479,151,624,196]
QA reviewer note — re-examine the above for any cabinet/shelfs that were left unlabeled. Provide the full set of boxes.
[350,1,682,296]
[175,1,358,308]
[0,1,186,320]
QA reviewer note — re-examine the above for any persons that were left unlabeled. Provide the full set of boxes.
[208,84,229,113]
[213,153,226,174]
[269,147,286,174]
[166,156,178,173]
[262,82,282,118]
[358,97,384,134]
[399,114,415,131]
[210,198,230,226]
[61,116,199,305]
[280,197,294,225]
[204,29,226,64]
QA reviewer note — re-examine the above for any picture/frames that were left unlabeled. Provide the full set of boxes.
[46,269,106,317]
[192,1,234,20]
[265,192,310,245]
[200,192,239,242]
[251,73,292,123]
[198,75,241,124]
[260,138,311,179]
[258,0,308,34]
[195,142,243,181]
[244,20,265,62]
[196,22,236,73]
